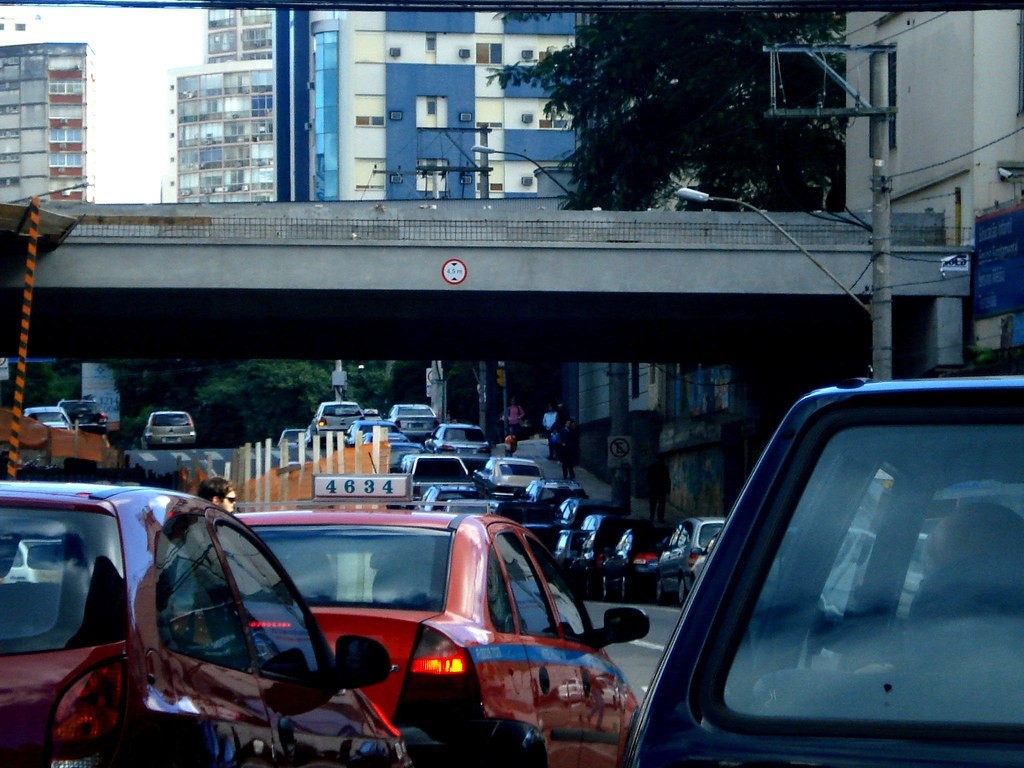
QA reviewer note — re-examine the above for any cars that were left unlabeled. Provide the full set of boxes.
[600,527,672,605]
[475,456,541,498]
[23,406,73,431]
[0,479,416,767]
[232,475,653,767]
[419,477,656,601]
[345,418,470,509]
[819,527,932,621]
[652,515,780,610]
[382,404,440,443]
[422,422,491,475]
[141,410,196,450]
[618,375,1023,768]
[277,429,307,448]
[310,401,365,448]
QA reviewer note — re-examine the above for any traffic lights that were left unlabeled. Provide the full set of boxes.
[497,369,504,386]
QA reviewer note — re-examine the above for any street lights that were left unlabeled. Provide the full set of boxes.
[675,187,894,384]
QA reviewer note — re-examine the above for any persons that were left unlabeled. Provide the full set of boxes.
[506,396,525,441]
[542,402,577,480]
[641,453,672,523]
[197,477,236,512]
[922,502,1024,590]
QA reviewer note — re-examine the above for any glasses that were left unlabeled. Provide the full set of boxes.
[224,497,238,503]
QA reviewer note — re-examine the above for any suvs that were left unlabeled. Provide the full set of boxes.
[56,398,109,438]
